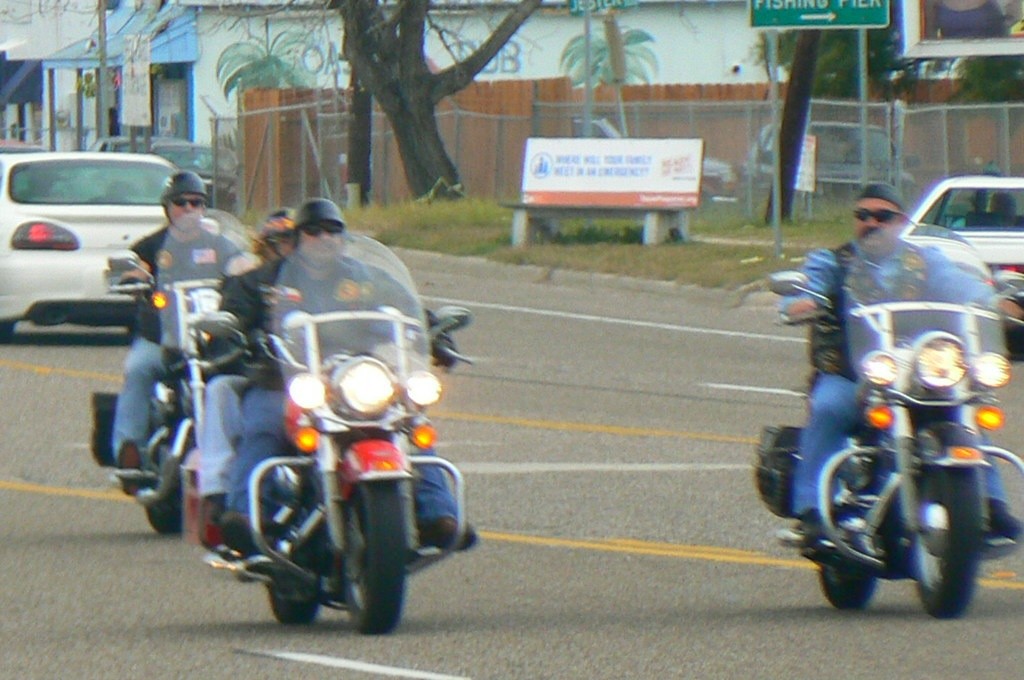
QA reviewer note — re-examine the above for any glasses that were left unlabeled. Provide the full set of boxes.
[856,208,899,221]
[171,196,202,207]
[305,223,343,237]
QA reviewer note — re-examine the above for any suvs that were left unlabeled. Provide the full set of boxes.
[740,122,922,215]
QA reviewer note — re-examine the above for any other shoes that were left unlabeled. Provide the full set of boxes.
[120,441,142,496]
[779,516,821,541]
[989,506,1024,538]
[419,516,476,551]
[220,514,261,560]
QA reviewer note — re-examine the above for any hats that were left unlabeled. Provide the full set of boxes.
[862,183,906,208]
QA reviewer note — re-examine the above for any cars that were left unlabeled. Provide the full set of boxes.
[0,135,239,344]
[571,112,739,207]
[897,175,1024,354]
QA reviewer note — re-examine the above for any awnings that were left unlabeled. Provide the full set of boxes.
[0,61,42,113]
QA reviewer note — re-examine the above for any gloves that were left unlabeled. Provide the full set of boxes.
[433,337,458,367]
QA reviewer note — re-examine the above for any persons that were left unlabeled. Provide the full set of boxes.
[194,197,477,555]
[925,0,1024,41]
[776,180,1024,548]
[111,168,243,495]
[109,106,120,136]
[956,190,1021,228]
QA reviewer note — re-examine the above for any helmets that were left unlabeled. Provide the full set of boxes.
[264,208,298,240]
[294,198,346,228]
[161,171,207,200]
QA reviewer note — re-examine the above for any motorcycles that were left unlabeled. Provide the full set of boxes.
[753,222,1024,620]
[91,208,255,535]
[187,233,479,634]
